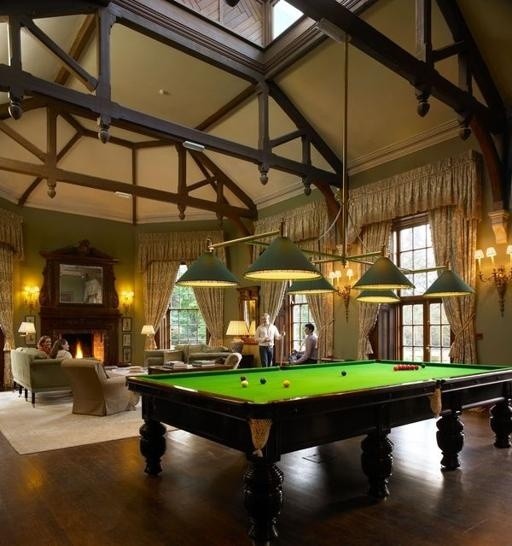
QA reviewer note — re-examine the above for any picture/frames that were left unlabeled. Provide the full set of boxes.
[25,315,36,345]
[122,317,133,362]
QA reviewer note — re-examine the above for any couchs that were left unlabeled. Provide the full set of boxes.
[10,343,243,416]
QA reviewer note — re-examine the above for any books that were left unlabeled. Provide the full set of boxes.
[191,360,217,368]
[164,360,189,370]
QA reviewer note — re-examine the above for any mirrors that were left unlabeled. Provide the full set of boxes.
[59,264,103,305]
[237,286,260,345]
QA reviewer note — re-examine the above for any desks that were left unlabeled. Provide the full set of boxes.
[125,357,512,545]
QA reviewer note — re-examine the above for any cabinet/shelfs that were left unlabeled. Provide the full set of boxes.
[239,355,255,367]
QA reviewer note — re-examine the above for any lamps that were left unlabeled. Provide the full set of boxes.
[18,322,37,336]
[328,269,353,323]
[226,320,249,343]
[174,33,476,303]
[113,191,132,199]
[249,321,256,336]
[474,245,512,318]
[119,291,134,315]
[25,285,40,315]
[183,140,205,153]
[140,325,156,351]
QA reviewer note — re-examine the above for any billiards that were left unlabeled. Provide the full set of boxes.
[398,366,402,370]
[394,366,398,370]
[402,365,406,369]
[241,376,246,380]
[260,378,266,384]
[283,380,290,387]
[241,381,248,387]
[406,365,410,369]
[415,365,419,369]
[341,371,347,376]
[421,364,425,368]
[411,366,415,370]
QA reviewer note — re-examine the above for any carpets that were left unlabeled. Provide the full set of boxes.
[1,388,180,455]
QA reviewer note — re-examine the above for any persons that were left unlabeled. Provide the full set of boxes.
[291,324,317,365]
[256,313,286,366]
[39,335,52,353]
[52,339,72,360]
